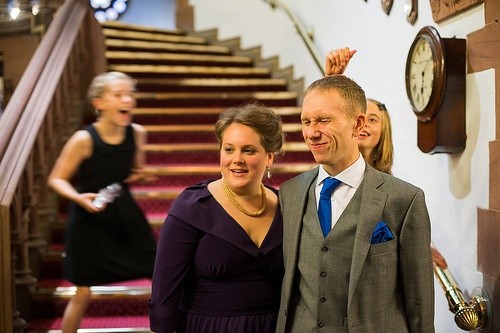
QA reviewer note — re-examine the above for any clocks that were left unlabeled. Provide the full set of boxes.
[404,25,468,155]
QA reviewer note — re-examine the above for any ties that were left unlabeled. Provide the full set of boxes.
[318,177,342,239]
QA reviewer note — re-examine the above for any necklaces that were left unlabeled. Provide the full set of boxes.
[221,177,267,216]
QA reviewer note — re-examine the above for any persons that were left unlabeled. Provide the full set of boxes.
[148,103,285,333]
[277,74,435,333]
[47,69,160,333]
[325,46,449,269]
